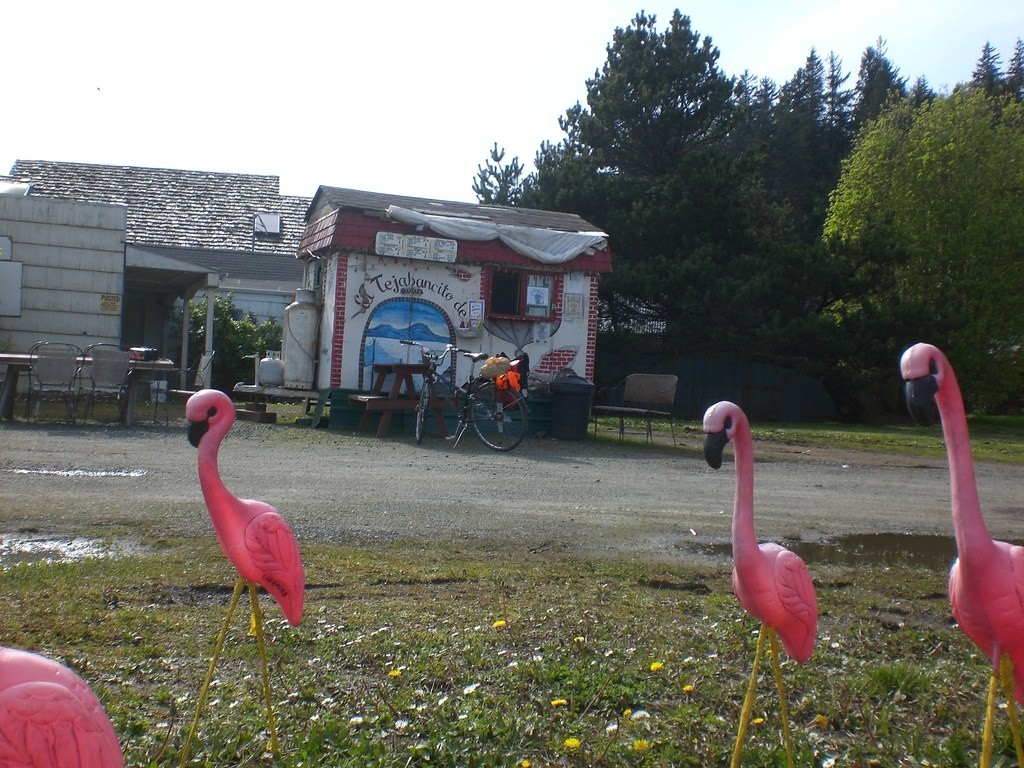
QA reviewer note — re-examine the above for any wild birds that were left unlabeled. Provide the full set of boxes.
[181,388,306,768]
[899,340,1024,768]
[702,401,819,768]
[0,645,126,768]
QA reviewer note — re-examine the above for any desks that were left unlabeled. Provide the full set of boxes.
[358,364,447,439]
[0,353,174,426]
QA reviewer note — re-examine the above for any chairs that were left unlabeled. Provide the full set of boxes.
[27,340,85,426]
[71,342,138,428]
[151,350,215,430]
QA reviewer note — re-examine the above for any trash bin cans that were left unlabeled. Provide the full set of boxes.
[548,374,598,442]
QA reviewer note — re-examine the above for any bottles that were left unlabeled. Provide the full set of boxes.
[460,320,471,328]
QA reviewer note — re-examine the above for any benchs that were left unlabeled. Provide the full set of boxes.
[348,394,389,409]
[420,395,459,410]
[593,373,679,448]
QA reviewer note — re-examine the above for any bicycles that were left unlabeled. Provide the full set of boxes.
[400,340,530,453]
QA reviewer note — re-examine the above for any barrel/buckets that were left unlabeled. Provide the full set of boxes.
[550,375,595,441]
